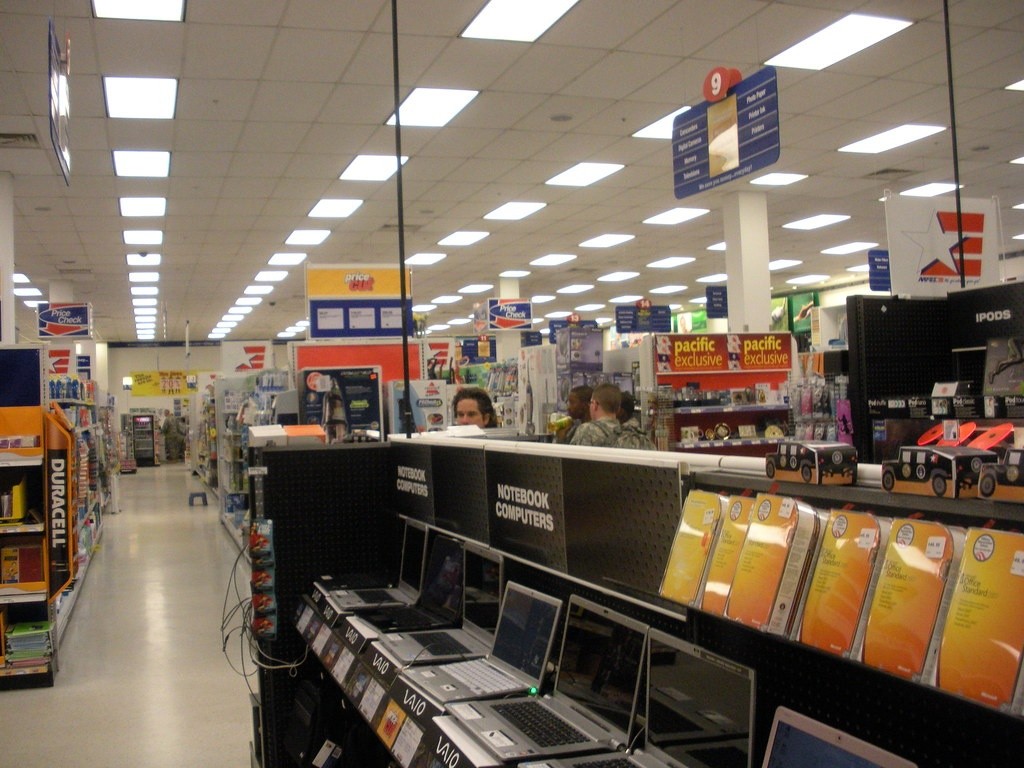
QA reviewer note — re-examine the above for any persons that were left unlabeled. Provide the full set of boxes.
[452,388,497,429]
[160,410,188,462]
[551,382,658,451]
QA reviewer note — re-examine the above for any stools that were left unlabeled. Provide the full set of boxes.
[188,492,207,507]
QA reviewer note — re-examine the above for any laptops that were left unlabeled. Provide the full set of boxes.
[313,508,918,768]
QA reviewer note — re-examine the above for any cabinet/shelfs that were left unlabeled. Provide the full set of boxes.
[649,401,799,456]
[0,398,110,688]
[182,374,285,507]
